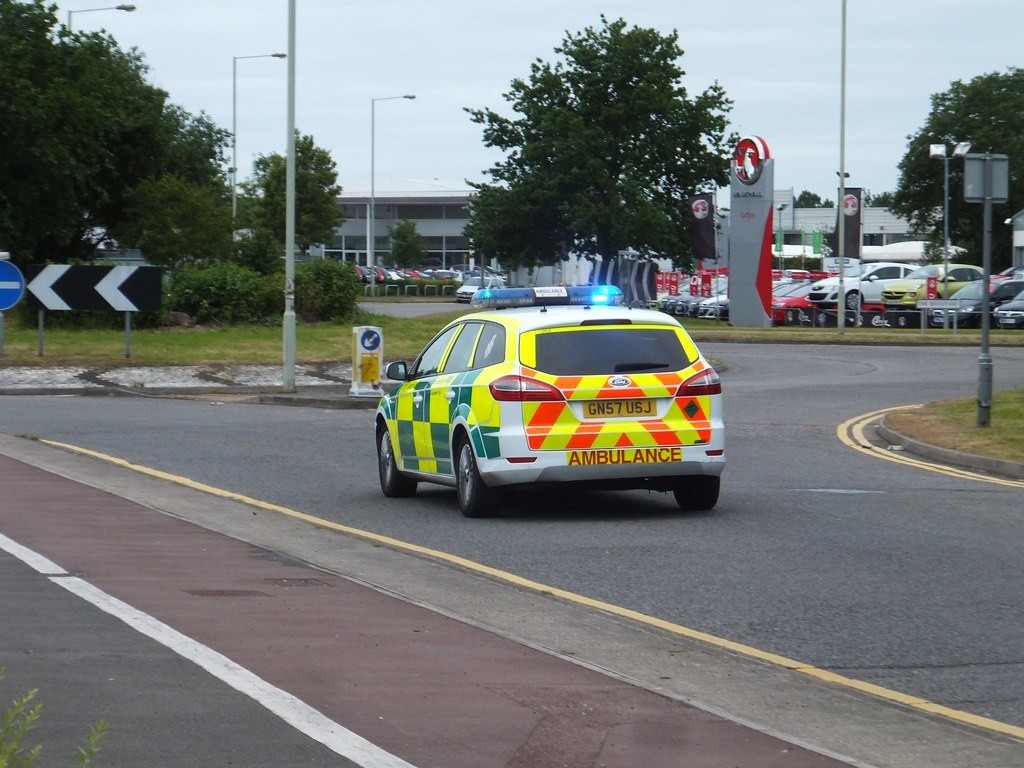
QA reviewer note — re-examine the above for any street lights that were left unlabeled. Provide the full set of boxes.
[776,202,789,269]
[370,94,416,266]
[928,141,971,331]
[64,4,137,36]
[232,52,289,222]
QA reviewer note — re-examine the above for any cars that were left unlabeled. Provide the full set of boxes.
[455,277,506,303]
[658,261,1024,329]
[354,264,507,284]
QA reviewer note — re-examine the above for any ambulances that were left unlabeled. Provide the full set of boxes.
[374,284,728,518]
